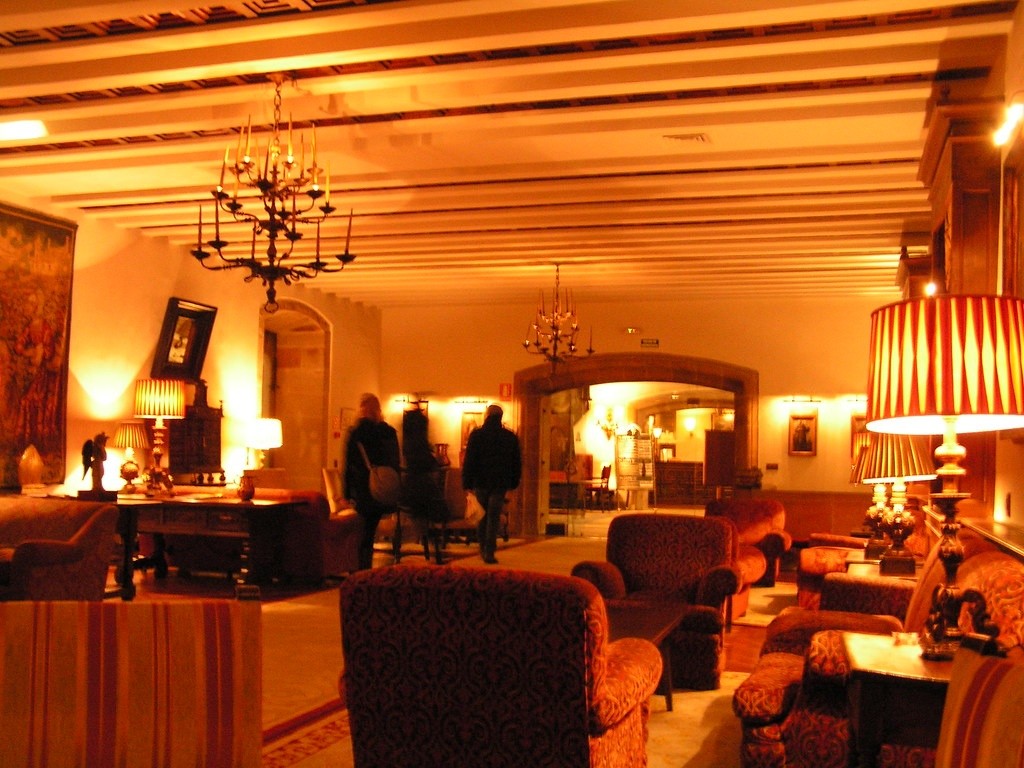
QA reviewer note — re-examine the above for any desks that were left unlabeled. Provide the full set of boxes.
[607,606,691,710]
[119,492,310,600]
[548,479,607,509]
[49,493,163,601]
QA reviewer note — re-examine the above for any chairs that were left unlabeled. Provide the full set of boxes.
[321,468,513,546]
[584,464,613,513]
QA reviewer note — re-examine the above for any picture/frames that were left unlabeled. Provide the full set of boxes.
[850,413,869,456]
[341,407,356,429]
[149,298,219,384]
[788,415,817,457]
[0,199,78,493]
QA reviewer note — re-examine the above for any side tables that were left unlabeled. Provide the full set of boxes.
[847,563,924,582]
[851,524,874,539]
[845,550,924,568]
[840,631,960,768]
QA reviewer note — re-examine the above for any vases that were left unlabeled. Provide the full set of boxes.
[236,475,255,501]
[435,443,451,469]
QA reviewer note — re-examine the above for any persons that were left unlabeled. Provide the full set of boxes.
[343,393,401,570]
[461,405,522,564]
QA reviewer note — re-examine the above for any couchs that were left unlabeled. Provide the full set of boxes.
[734,489,893,561]
[733,507,1024,768]
[572,514,743,693]
[0,492,120,603]
[337,562,664,768]
[705,495,792,587]
[0,600,262,768]
[129,481,358,589]
[706,515,766,633]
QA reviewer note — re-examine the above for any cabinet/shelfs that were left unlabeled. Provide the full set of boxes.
[655,460,703,508]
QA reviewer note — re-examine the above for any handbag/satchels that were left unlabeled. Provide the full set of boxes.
[465,493,485,525]
[369,466,401,506]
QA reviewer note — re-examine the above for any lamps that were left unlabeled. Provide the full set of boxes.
[110,420,151,494]
[134,377,185,494]
[850,445,891,560]
[246,418,283,470]
[867,292,1024,659]
[522,261,595,381]
[654,427,662,439]
[189,69,358,314]
[863,430,937,576]
[684,417,694,439]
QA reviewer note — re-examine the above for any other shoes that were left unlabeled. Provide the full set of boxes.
[482,551,498,564]
